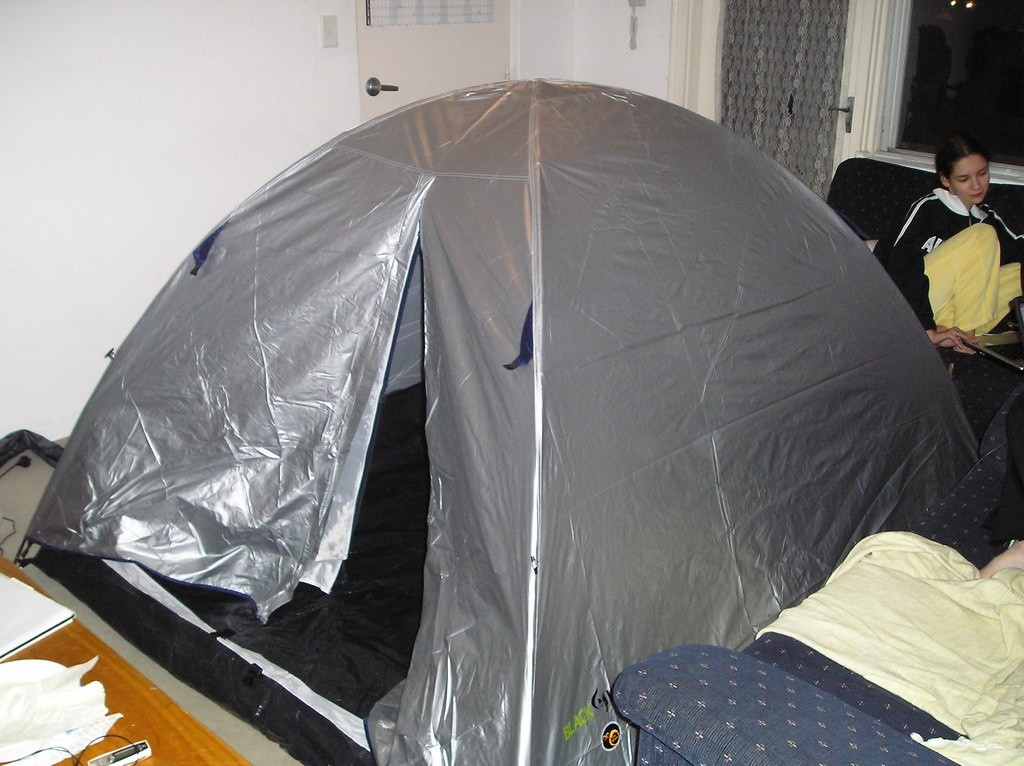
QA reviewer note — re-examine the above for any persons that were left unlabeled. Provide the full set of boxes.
[872,136,1024,354]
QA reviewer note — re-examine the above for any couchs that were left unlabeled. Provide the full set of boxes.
[826,158,1024,443]
[612,384,1024,766]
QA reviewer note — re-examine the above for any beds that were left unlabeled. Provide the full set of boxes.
[35,383,430,765]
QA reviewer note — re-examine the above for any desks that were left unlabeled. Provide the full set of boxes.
[0,555,253,766]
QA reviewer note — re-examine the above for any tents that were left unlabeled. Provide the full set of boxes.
[14,78,983,766]
